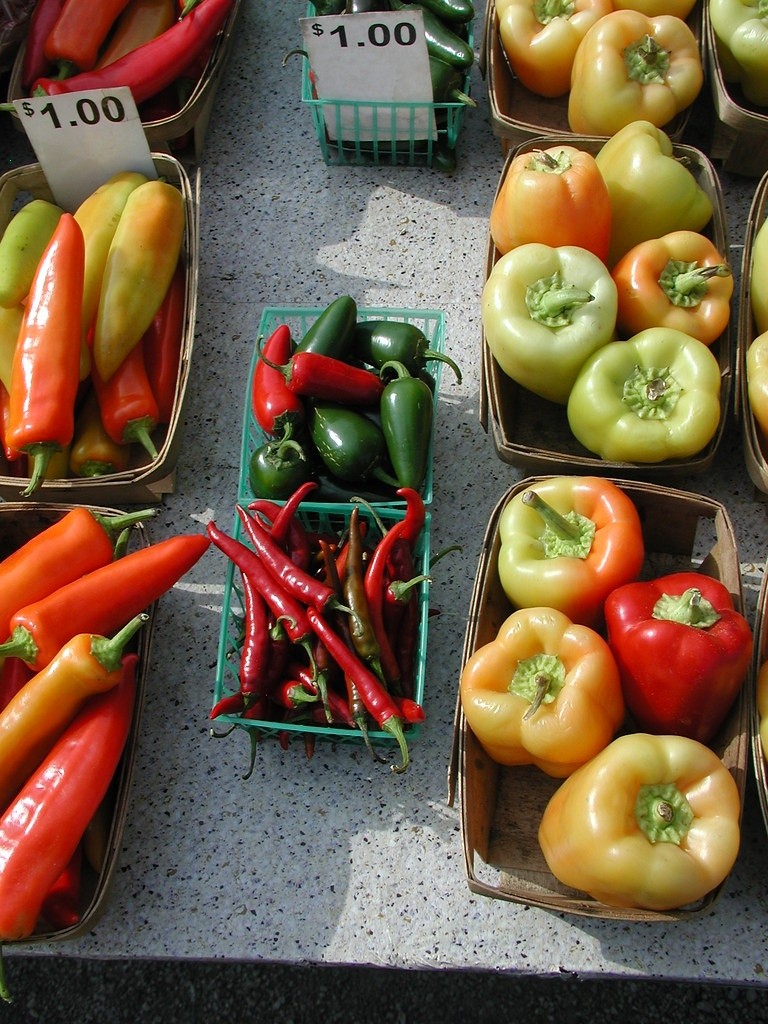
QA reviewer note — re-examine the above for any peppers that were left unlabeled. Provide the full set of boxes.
[0,0,231,150]
[461,476,768,913]
[247,294,462,503]
[280,0,478,173]
[202,481,464,777]
[0,507,214,1005]
[478,120,768,465]
[0,172,189,498]
[493,0,768,136]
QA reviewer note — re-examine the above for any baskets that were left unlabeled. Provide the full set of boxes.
[445,474,750,919]
[481,0,707,143]
[0,503,156,941]
[476,135,736,471]
[709,12,767,133]
[738,171,768,497]
[7,1,239,159]
[1,151,202,500]
[304,0,473,170]
[216,308,444,737]
[750,563,768,826]
